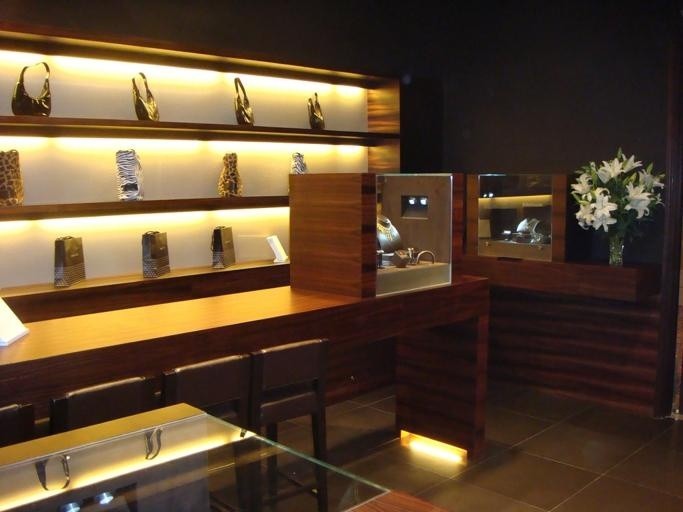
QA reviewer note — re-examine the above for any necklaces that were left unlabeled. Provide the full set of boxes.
[377,219,393,241]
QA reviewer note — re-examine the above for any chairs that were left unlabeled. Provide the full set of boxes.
[2,405,37,445]
[163,354,252,504]
[49,375,150,425]
[251,339,331,506]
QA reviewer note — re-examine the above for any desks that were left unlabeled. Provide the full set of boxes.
[0,269,492,454]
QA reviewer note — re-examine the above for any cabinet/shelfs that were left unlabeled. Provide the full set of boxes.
[0,31,406,324]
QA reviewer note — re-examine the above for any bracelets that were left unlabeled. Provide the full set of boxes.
[417,250,435,264]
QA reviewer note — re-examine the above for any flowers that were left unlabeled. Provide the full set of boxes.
[571,146,667,263]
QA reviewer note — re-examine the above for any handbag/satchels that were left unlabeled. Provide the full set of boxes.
[12,79,51,116]
[234,94,255,126]
[212,226,236,269]
[133,89,159,122]
[54,237,86,287]
[116,149,144,200]
[0,150,23,207]
[142,231,170,279]
[292,154,310,174]
[219,153,244,197]
[308,101,325,128]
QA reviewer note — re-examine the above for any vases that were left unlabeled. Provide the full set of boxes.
[607,237,624,267]
